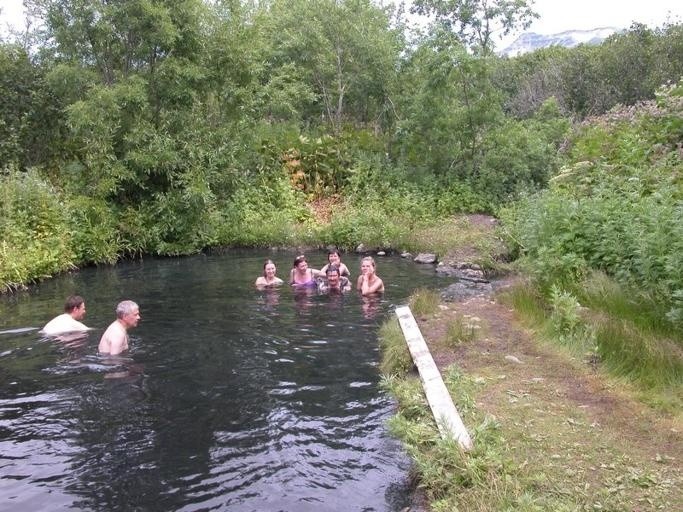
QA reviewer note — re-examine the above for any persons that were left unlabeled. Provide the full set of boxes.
[255,259,283,284]
[41,295,92,334]
[289,255,346,287]
[356,256,384,296]
[316,264,347,295]
[320,252,351,275]
[97,300,140,356]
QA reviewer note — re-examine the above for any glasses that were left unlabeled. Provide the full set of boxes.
[296,255,304,261]
